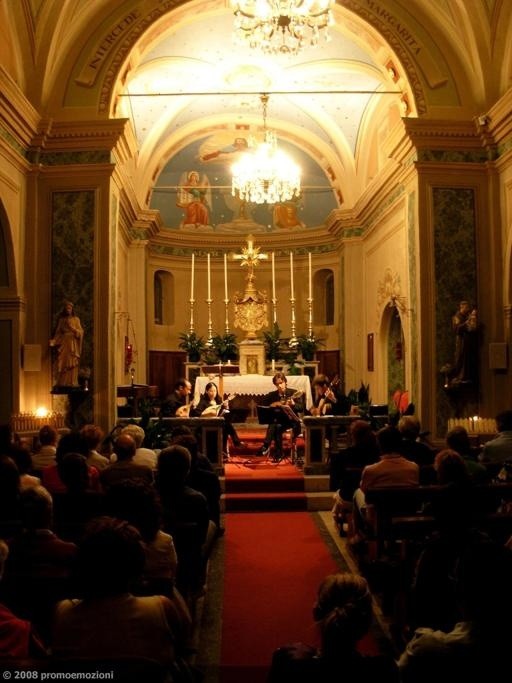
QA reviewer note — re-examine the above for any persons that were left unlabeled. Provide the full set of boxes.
[196,383,245,452]
[48,301,84,388]
[3,423,222,683]
[308,373,351,464]
[269,190,307,232]
[451,300,477,384]
[177,170,213,233]
[158,377,192,418]
[268,410,511,683]
[257,374,306,458]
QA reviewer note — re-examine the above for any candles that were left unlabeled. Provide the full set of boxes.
[190,253,196,302]
[207,253,212,300]
[308,253,312,297]
[290,252,294,298]
[448,415,497,432]
[272,252,275,300]
[224,254,229,299]
[272,359,275,371]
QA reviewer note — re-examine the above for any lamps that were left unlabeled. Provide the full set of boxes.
[231,94,302,205]
[232,1,335,55]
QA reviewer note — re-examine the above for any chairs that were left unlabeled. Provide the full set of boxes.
[340,456,512,683]
[271,393,307,463]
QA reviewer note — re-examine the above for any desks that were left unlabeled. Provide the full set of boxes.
[117,384,161,403]
[193,376,315,410]
[183,361,321,405]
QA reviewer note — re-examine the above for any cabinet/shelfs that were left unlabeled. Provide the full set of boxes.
[302,415,390,475]
[117,416,226,478]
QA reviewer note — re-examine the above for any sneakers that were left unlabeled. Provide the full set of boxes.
[273,453,282,462]
[256,446,270,456]
[235,441,248,448]
[223,453,231,463]
